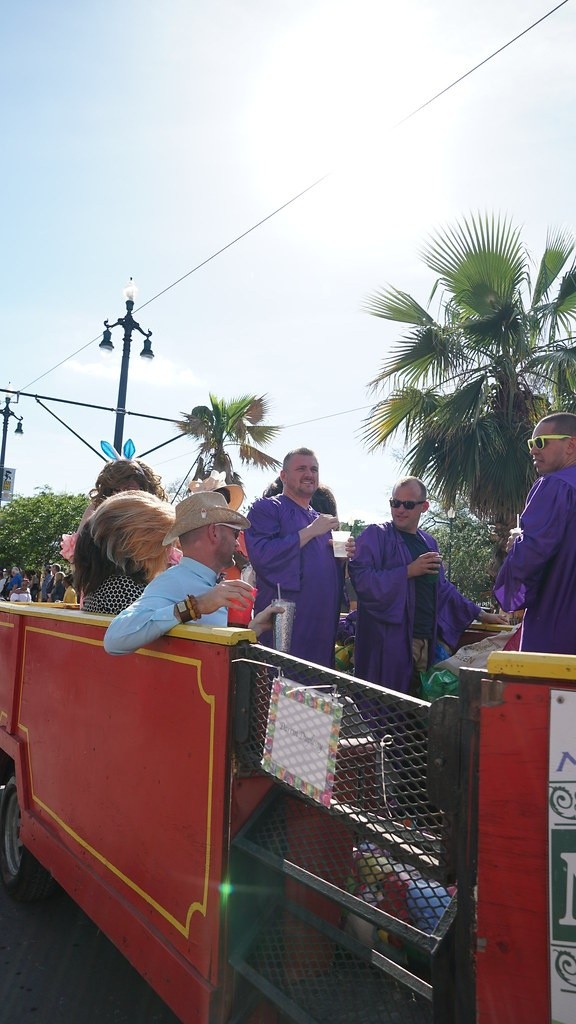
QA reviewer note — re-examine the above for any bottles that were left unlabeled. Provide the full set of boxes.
[425,546,440,583]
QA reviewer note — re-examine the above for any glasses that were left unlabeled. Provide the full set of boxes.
[21,583,28,586]
[527,434,571,450]
[222,525,241,539]
[389,497,426,510]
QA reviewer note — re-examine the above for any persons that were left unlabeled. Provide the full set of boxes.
[245,448,355,693]
[42,564,77,604]
[348,476,508,824]
[495,412,575,655]
[76,460,337,656]
[0,567,39,602]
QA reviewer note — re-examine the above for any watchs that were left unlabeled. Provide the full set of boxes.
[176,600,192,623]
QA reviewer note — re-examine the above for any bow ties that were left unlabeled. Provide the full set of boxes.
[216,572,227,584]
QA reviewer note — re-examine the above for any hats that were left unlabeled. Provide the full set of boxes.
[42,564,52,570]
[162,491,251,547]
[49,563,63,571]
[188,470,245,511]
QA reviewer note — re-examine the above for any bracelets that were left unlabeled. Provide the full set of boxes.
[185,596,197,621]
[190,595,201,619]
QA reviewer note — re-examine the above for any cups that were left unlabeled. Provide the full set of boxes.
[271,598,296,653]
[331,530,351,558]
[227,587,258,628]
[509,527,525,545]
